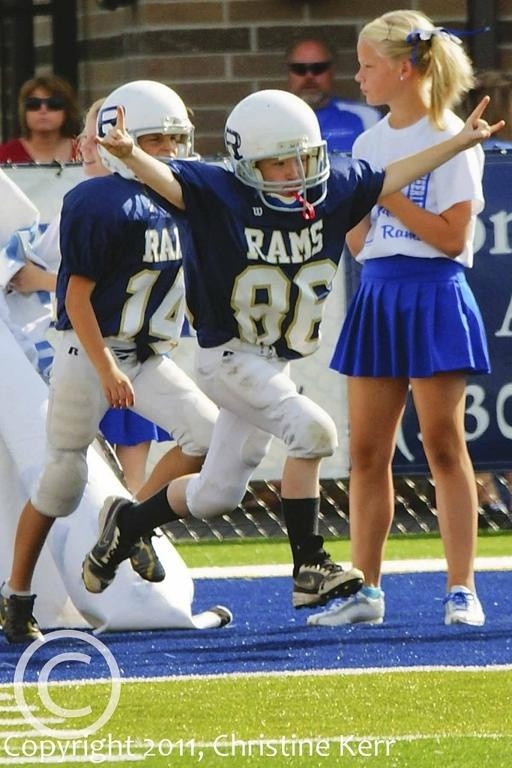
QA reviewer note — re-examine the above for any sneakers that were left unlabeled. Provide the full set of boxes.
[0,580,46,644]
[305,588,385,627]
[291,535,365,611]
[443,587,486,627]
[130,530,167,582]
[81,495,142,593]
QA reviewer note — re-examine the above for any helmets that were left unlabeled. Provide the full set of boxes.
[222,87,323,188]
[94,80,193,186]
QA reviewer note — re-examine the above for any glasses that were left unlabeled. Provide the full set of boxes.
[23,95,67,112]
[285,60,332,77]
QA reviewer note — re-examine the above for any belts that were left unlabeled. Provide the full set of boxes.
[226,337,279,359]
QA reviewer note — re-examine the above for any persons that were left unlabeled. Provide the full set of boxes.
[289,40,383,159]
[0,80,220,648]
[307,7,487,627]
[1,75,84,164]
[84,88,505,607]
[8,95,192,497]
